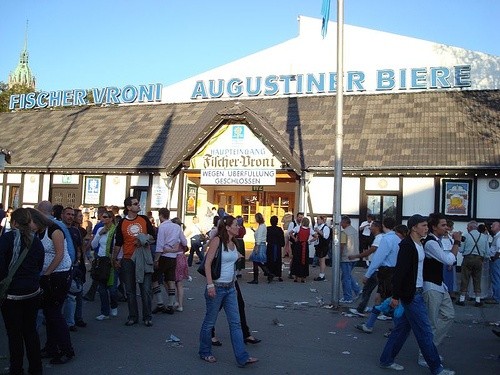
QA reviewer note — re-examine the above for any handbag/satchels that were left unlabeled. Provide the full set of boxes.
[197,237,222,280]
[89,256,110,282]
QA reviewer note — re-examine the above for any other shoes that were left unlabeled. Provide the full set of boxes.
[70,326,77,330]
[437,369,455,375]
[339,290,497,337]
[125,320,138,326]
[246,357,259,363]
[77,320,87,327]
[40,348,55,358]
[418,355,430,367]
[268,274,328,283]
[111,308,118,317]
[177,307,183,312]
[152,306,165,313]
[96,314,110,320]
[379,362,404,371]
[50,352,72,364]
[162,309,174,314]
[201,355,217,362]
[144,320,154,326]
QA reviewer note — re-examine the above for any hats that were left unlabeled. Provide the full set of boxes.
[406,214,430,229]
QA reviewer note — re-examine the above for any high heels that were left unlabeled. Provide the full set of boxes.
[212,340,222,346]
[244,338,262,344]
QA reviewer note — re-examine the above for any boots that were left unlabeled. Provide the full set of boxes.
[251,273,258,284]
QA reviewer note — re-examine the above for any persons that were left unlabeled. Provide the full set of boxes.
[378,213,457,375]
[197,215,259,367]
[0,197,500,375]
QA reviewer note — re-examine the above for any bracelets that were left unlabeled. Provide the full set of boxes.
[454,240,459,246]
[207,284,214,289]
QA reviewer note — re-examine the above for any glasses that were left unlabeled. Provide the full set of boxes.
[103,216,107,218]
[132,203,137,205]
